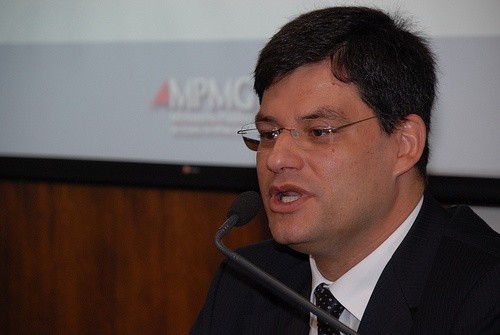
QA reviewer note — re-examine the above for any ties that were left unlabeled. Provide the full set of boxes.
[315,283,346,334]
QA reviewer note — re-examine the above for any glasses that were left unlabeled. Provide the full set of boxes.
[238,114,378,153]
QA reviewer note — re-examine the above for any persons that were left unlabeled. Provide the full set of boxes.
[188,6,500,335]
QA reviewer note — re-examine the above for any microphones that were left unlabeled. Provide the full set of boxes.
[214,192,360,335]
